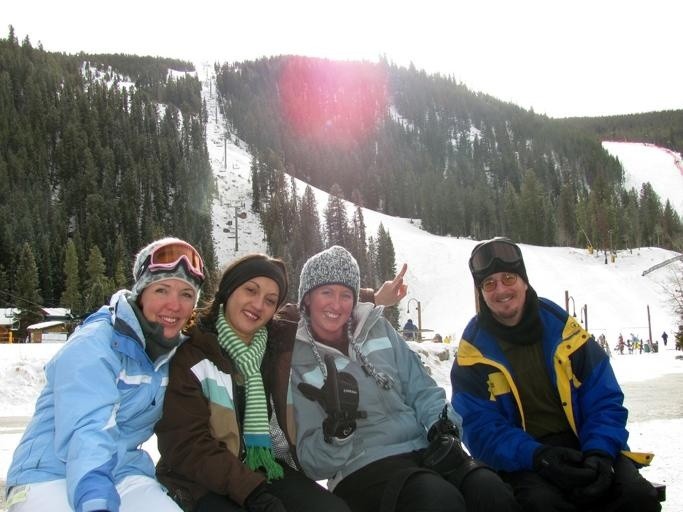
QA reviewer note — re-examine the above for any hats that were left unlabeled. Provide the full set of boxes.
[129,235,207,312]
[467,236,530,290]
[296,244,361,312]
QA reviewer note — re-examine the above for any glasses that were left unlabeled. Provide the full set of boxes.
[468,240,521,274]
[132,240,206,289]
[477,272,518,293]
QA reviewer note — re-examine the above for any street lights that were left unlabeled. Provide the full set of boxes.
[407,298,422,343]
[565,290,576,317]
[581,304,587,331]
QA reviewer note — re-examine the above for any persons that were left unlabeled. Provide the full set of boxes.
[284,242,527,512]
[591,332,640,356]
[431,333,451,344]
[151,252,409,512]
[0,234,209,511]
[446,234,668,512]
[660,331,668,346]
[402,319,418,341]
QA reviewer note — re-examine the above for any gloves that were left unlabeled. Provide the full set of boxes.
[296,353,367,446]
[245,486,288,512]
[420,404,464,473]
[530,443,616,502]
